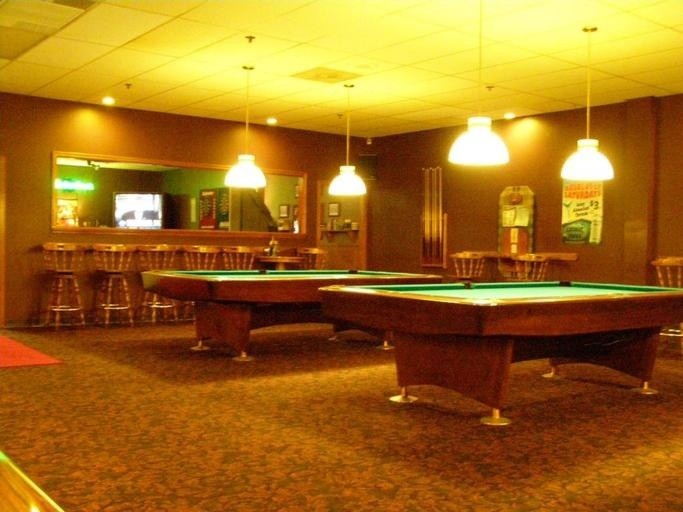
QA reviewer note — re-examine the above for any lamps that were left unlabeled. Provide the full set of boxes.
[223,65,267,188]
[447,3,509,167]
[328,83,370,197]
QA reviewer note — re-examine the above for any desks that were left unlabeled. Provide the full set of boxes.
[317,280,683,427]
[138,268,442,363]
[257,255,305,271]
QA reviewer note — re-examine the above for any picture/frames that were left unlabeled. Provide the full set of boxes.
[279,205,289,218]
[327,203,340,217]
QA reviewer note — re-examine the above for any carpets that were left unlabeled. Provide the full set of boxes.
[0,335,63,367]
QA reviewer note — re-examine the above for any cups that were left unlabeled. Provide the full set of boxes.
[269,241,279,257]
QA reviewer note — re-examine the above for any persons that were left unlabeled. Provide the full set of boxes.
[120,197,159,219]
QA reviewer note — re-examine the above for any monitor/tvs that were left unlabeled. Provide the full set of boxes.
[112,190,168,229]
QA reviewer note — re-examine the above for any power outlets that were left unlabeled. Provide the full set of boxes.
[559,26,614,182]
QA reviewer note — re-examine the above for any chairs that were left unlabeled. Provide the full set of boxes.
[450,251,486,282]
[41,241,257,328]
[295,249,326,269]
[650,255,683,360]
[511,254,551,281]
